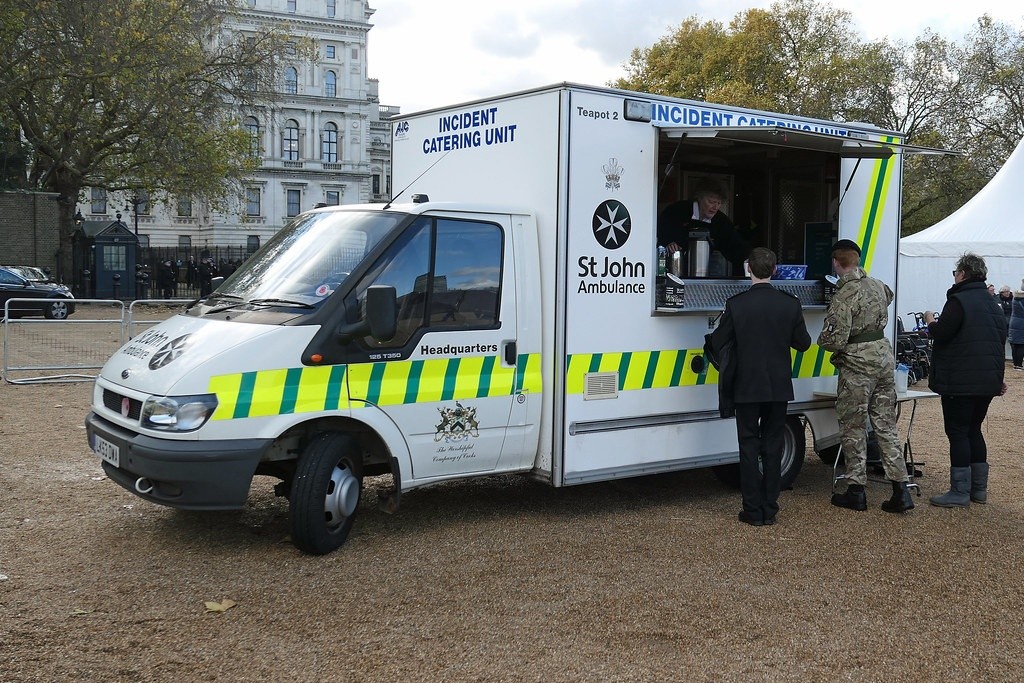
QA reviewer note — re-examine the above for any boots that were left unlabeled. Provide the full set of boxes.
[832,484,867,511]
[882,481,915,512]
[930,466,972,508]
[970,462,989,504]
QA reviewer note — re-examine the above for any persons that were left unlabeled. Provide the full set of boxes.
[156,256,217,299]
[817,239,914,513]
[985,280,1024,371]
[709,248,811,527]
[658,174,751,267]
[923,252,1007,508]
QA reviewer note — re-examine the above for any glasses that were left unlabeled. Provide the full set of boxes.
[953,271,958,276]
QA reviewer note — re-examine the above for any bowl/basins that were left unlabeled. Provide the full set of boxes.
[772,265,808,280]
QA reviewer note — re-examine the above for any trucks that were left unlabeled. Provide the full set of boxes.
[83,78,962,558]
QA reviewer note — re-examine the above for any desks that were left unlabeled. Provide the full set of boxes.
[813,391,940,494]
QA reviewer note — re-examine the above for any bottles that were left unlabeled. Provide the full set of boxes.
[658,246,666,276]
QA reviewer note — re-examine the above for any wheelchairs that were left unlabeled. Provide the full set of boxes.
[897,311,941,388]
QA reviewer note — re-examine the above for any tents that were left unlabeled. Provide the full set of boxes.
[900,137,1024,360]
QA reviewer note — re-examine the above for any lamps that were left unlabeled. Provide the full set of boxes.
[73,209,85,228]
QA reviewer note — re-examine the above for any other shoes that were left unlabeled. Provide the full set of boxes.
[764,519,776,525]
[1013,365,1024,371]
[739,511,764,526]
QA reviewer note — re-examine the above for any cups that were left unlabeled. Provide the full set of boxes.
[667,250,683,277]
[895,371,908,393]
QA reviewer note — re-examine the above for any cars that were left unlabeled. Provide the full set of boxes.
[0,266,75,321]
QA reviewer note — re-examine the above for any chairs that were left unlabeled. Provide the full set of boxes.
[363,237,482,324]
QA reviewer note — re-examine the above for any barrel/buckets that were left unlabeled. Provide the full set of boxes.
[687,240,710,277]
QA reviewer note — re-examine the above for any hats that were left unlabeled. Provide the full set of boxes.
[832,239,861,257]
[986,282,994,288]
[1020,279,1024,291]
[998,285,1010,292]
